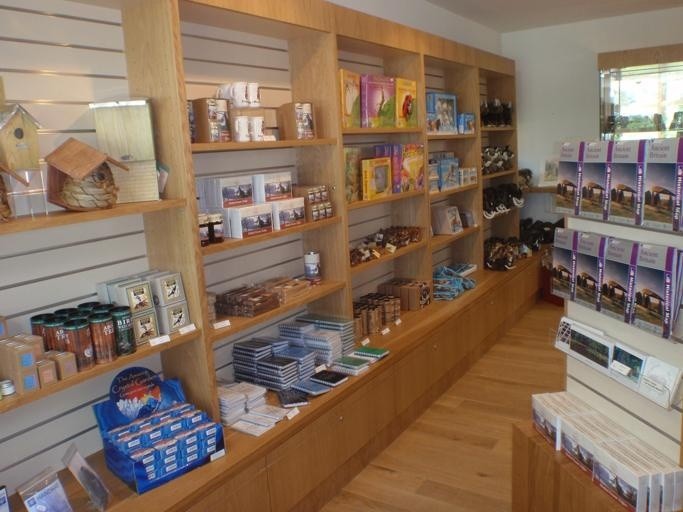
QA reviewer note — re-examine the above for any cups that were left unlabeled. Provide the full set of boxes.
[301,249,322,281]
[217,83,229,100]
[233,116,249,141]
[250,117,264,141]
[247,83,259,107]
[230,82,246,106]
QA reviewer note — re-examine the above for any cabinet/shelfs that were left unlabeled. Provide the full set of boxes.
[511,417,682,512]
[0,0,553,512]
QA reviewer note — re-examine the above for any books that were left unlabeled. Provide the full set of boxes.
[217,314,391,438]
[430,205,473,235]
[550,227,683,340]
[196,171,306,239]
[531,391,683,512]
[554,137,683,234]
